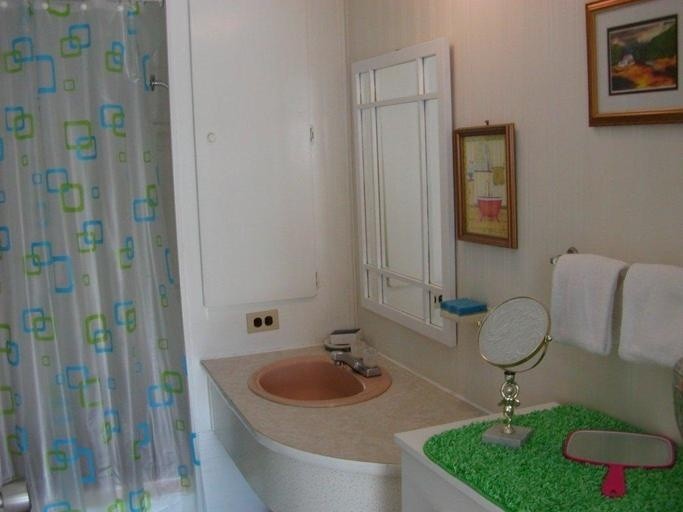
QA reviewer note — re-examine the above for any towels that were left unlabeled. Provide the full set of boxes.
[548,250,683,368]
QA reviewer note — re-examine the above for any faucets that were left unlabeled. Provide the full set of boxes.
[330,348,382,378]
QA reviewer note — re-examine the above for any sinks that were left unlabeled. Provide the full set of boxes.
[246,352,392,408]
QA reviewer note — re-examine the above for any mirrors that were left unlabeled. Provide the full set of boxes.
[565,432,674,496]
[478,297,552,447]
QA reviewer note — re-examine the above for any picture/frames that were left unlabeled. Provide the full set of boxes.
[582,0,683,127]
[451,121,520,254]
